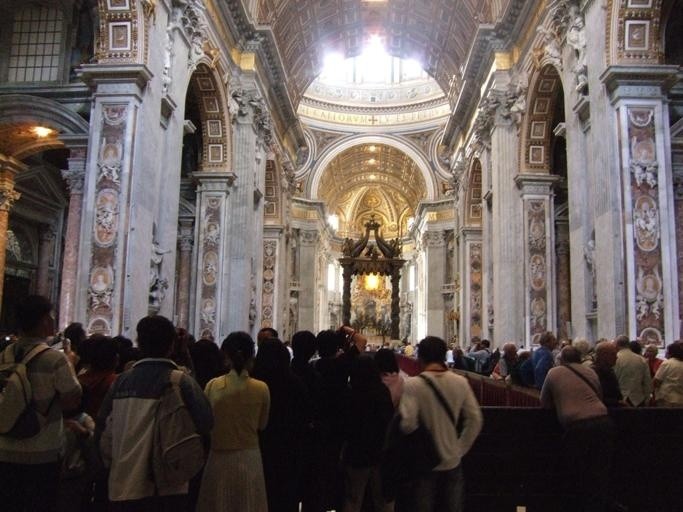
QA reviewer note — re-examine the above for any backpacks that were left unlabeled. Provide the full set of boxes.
[148,366,208,487]
[0,341,60,441]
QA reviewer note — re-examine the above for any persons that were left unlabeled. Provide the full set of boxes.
[1,295,682,511]
